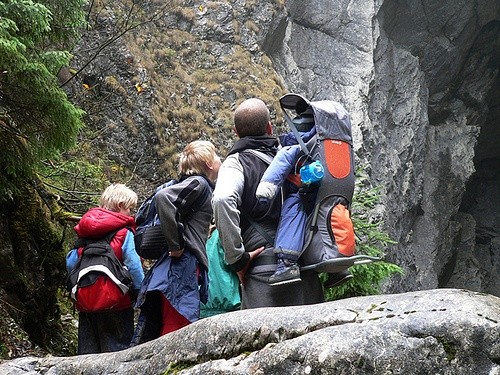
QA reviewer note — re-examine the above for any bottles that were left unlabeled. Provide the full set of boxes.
[300,161,324,184]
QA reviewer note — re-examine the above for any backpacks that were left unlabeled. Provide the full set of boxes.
[71,230,135,314]
[133,176,211,261]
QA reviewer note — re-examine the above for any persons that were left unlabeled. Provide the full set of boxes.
[64,184,145,356]
[143,138,223,339]
[255,107,355,289]
[208,98,326,310]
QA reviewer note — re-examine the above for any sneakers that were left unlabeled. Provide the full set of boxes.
[268,263,303,288]
[323,267,354,290]
